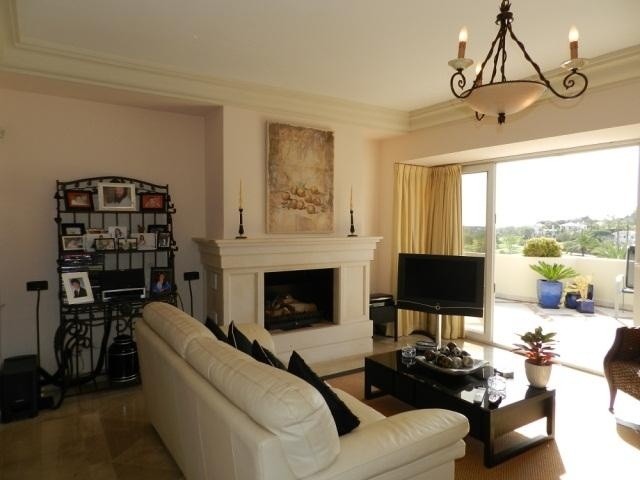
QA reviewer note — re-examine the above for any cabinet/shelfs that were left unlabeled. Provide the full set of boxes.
[36,173,179,399]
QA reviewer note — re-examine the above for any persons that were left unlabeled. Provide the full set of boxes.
[68,276,87,299]
[104,186,132,208]
[64,223,169,253]
[70,192,90,207]
[152,272,171,294]
[144,196,163,209]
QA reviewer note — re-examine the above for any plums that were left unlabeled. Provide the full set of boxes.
[425,341,474,368]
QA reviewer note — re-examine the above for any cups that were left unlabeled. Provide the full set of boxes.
[401,344,416,357]
[401,358,417,368]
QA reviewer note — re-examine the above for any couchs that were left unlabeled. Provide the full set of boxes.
[132,299,472,480]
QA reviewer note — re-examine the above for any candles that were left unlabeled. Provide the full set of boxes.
[350,185,354,211]
[238,177,244,211]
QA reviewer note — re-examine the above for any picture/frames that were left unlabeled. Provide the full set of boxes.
[97,181,138,213]
[62,188,95,213]
[138,191,167,215]
[61,269,96,306]
[60,218,171,257]
[150,264,174,299]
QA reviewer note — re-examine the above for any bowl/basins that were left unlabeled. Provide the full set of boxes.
[421,373,484,393]
[487,377,508,390]
[488,388,508,398]
[416,354,489,377]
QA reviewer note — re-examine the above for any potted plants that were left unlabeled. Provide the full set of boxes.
[511,326,562,389]
[528,259,581,309]
[564,274,594,313]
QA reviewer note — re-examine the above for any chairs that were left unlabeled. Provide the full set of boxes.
[614,245,635,321]
[603,325,640,413]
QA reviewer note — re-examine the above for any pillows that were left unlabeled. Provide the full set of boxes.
[250,338,286,372]
[229,319,252,356]
[287,350,361,437]
[204,315,229,347]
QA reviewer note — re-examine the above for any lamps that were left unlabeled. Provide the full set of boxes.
[447,0,592,126]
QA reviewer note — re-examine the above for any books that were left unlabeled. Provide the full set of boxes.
[370,300,393,308]
[371,293,394,301]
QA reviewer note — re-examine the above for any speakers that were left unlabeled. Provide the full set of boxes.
[0,354,39,424]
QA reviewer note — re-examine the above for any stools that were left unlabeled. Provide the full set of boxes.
[370,291,399,343]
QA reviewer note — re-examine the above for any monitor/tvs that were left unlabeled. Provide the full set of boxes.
[397,253,485,318]
